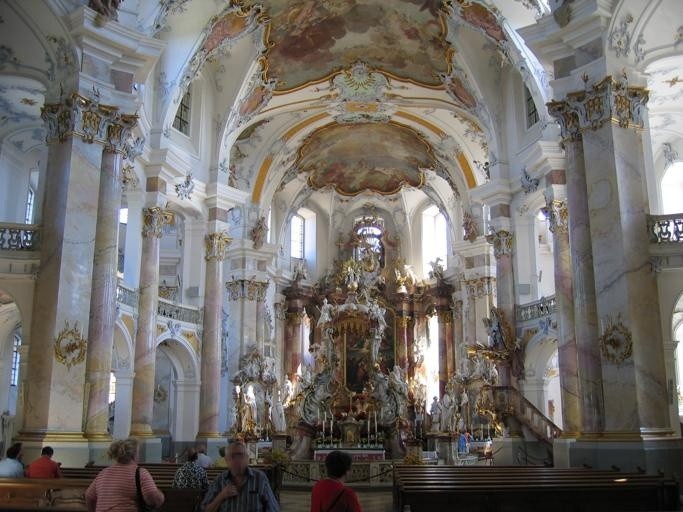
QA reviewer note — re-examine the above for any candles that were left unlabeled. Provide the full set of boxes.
[366,411,377,432]
[316,407,333,431]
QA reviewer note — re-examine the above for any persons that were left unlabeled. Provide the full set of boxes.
[0,445,24,478]
[191,445,213,467]
[232,344,313,434]
[310,449,364,512]
[462,212,479,241]
[197,442,280,512]
[211,445,230,468]
[253,216,269,245]
[426,304,508,433]
[25,445,64,478]
[12,442,25,465]
[84,436,166,511]
[456,429,470,453]
[314,263,388,372]
[170,446,211,488]
[317,154,428,194]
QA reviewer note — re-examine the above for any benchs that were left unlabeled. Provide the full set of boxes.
[388,460,683,512]
[0,462,277,512]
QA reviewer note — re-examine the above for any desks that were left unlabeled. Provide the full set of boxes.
[313,447,386,461]
